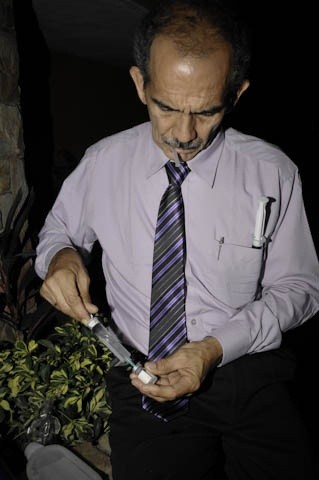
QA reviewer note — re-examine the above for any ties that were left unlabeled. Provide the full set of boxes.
[142,158,191,422]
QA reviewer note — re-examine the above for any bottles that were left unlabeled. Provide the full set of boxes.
[19,437,104,480]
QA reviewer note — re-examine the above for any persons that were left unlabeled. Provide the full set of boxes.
[32,0,319,480]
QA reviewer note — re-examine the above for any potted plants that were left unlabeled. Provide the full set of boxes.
[0,188,111,445]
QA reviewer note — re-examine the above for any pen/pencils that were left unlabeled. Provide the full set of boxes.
[217,237,224,260]
